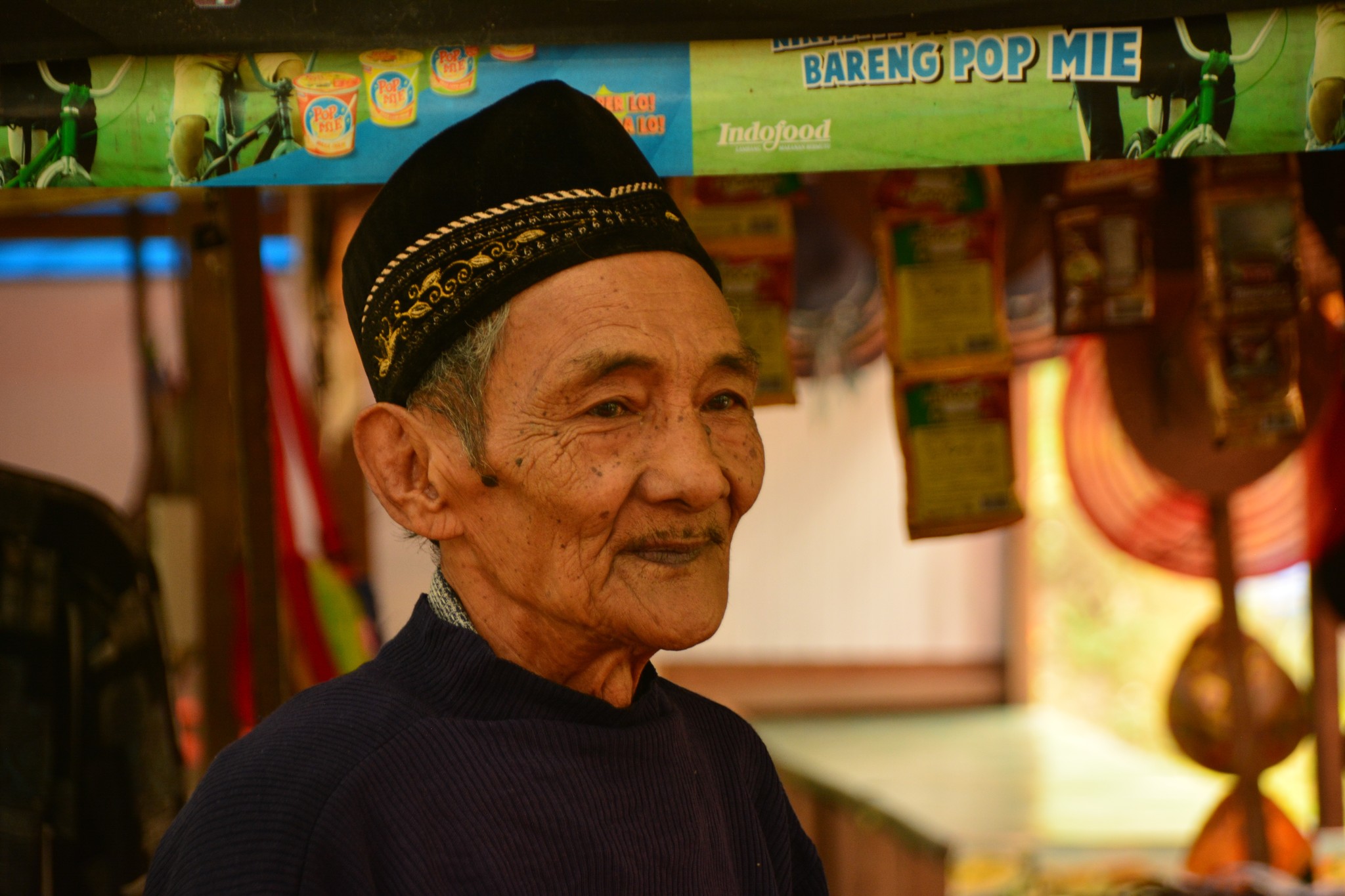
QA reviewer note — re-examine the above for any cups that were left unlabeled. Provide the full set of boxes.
[291,70,362,158]
[358,48,425,129]
[427,45,480,96]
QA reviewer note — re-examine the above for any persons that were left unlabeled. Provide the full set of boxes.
[1062,1,1345,161]
[170,54,309,184]
[140,75,834,896]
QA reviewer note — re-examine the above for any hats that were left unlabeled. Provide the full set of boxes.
[341,77,726,414]
